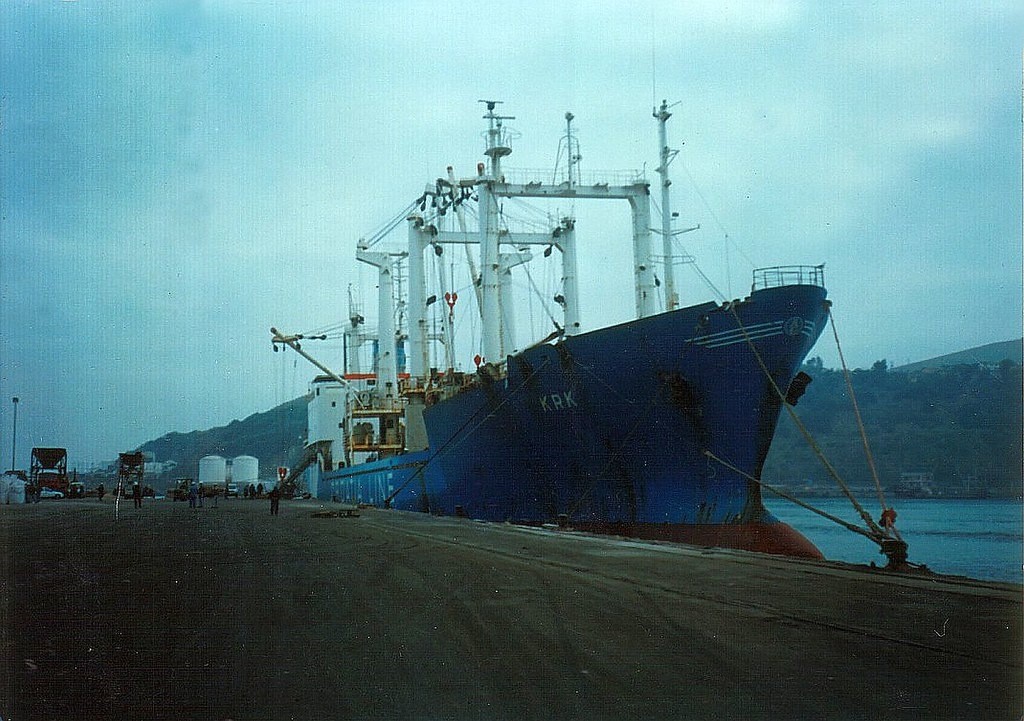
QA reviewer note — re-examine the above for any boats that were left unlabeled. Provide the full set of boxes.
[266,24,839,563]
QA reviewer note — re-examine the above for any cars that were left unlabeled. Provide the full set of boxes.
[39,486,65,500]
[227,483,240,497]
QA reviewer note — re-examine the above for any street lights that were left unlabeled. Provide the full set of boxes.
[11,397,20,470]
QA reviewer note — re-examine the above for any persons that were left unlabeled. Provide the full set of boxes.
[269,486,281,516]
[189,481,198,508]
[180,482,188,489]
[71,484,85,498]
[98,483,105,500]
[211,484,219,508]
[27,483,44,504]
[199,481,206,504]
[244,485,249,496]
[134,486,141,509]
[249,484,256,499]
[257,483,263,497]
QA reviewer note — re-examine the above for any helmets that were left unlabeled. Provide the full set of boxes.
[101,483,104,484]
[133,482,138,485]
[214,483,218,486]
[191,482,195,484]
[199,481,203,483]
